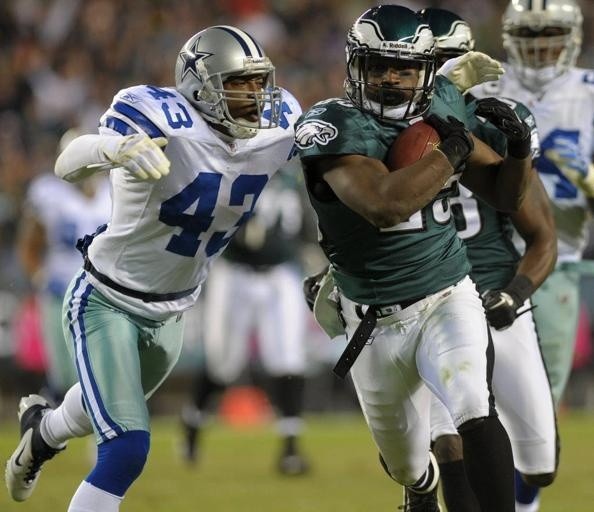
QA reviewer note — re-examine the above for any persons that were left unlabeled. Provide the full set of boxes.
[467,0,593,411]
[0,24,308,512]
[292,6,544,512]
[412,7,562,511]
[1,1,512,477]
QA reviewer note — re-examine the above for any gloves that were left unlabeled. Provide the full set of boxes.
[101,132,171,181]
[542,136,594,200]
[425,112,475,162]
[445,51,504,94]
[479,286,524,332]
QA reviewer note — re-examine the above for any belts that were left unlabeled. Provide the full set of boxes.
[330,292,427,381]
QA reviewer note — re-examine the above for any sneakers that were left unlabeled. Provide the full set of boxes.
[4,393,68,502]
[397,484,442,512]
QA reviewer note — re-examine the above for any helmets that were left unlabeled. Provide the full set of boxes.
[173,25,283,139]
[500,0,585,93]
[342,4,439,121]
[416,7,476,56]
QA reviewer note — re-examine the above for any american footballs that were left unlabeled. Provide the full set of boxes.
[386,119,441,171]
